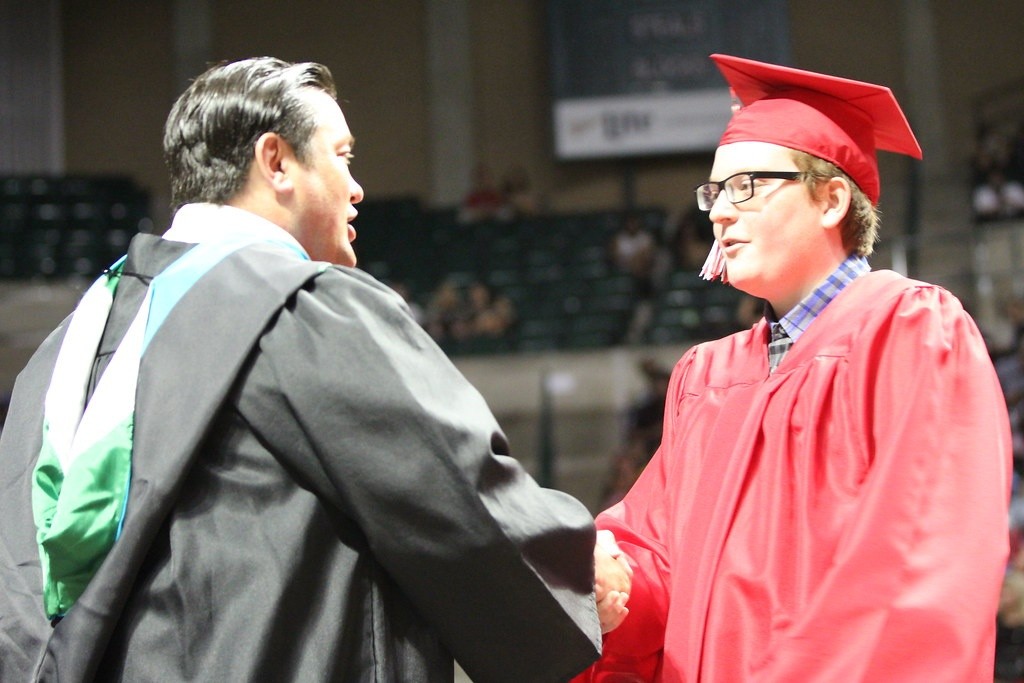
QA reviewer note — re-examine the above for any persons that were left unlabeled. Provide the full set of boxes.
[384,269,514,352]
[998,475,1024,627]
[455,169,545,222]
[0,58,628,683]
[574,53,1013,683]
[612,196,714,276]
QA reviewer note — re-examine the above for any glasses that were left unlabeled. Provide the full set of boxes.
[693,171,809,213]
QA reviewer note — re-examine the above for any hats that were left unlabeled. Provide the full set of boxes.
[699,52,924,282]
